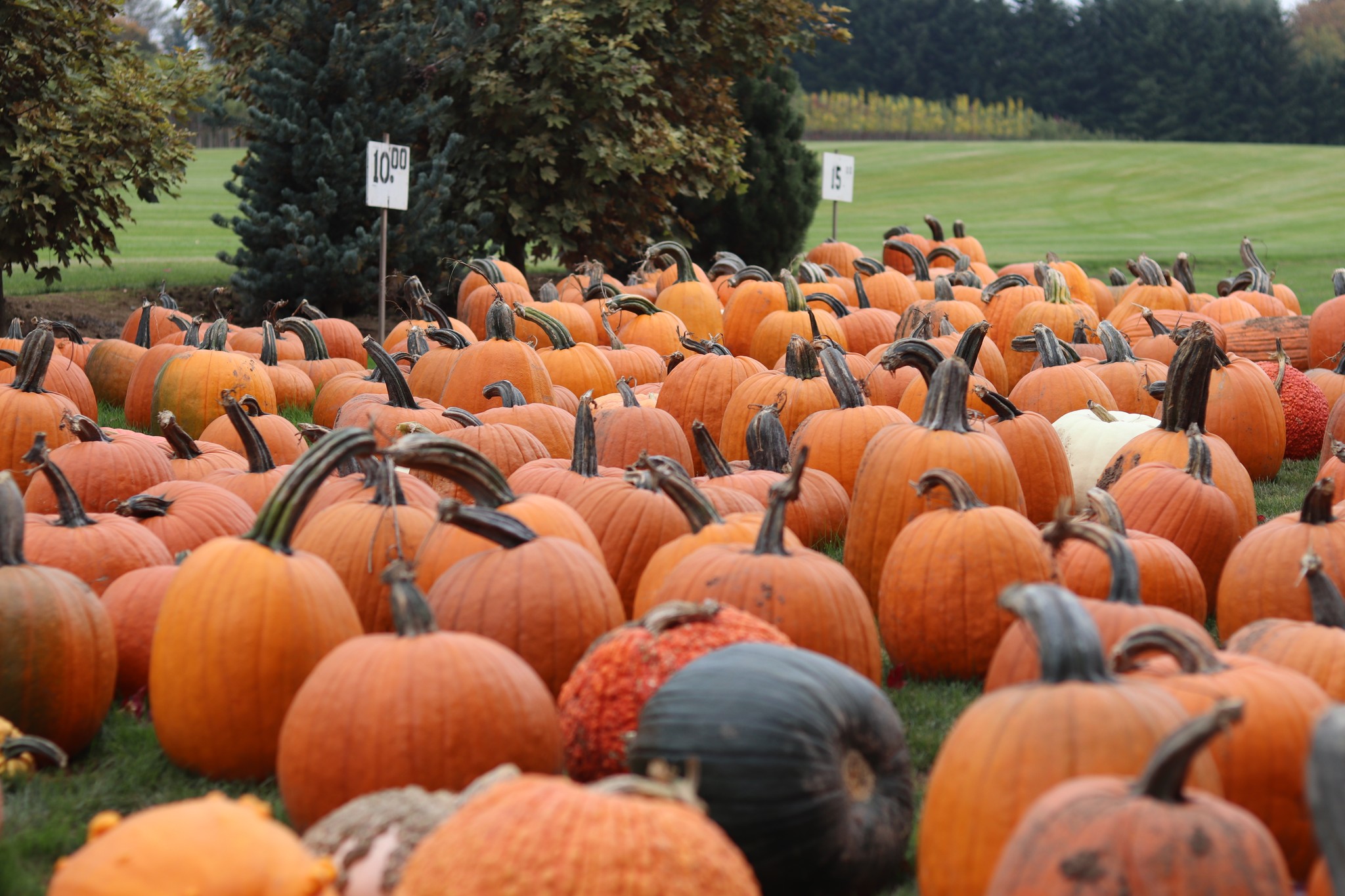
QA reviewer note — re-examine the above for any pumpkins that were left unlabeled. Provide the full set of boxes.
[1,213,1345,896]
